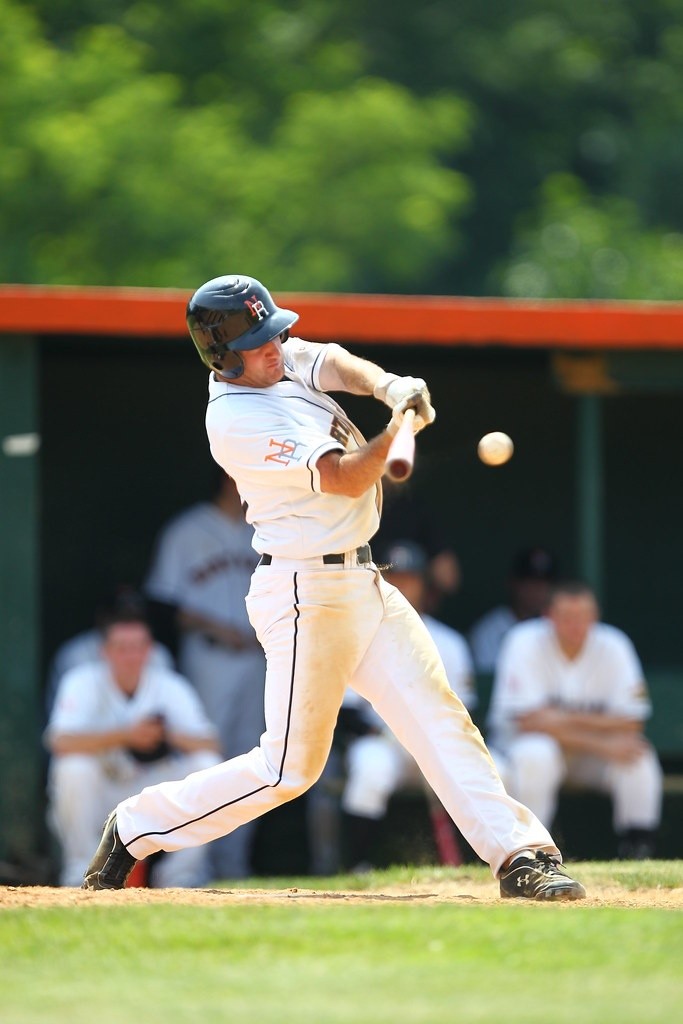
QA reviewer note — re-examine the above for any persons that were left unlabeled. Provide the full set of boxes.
[40,584,225,889]
[83,273,589,904]
[140,468,268,884]
[336,536,668,878]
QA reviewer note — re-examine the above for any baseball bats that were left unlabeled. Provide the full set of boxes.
[384,403,419,482]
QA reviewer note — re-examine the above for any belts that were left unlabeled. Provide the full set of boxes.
[260,544,374,566]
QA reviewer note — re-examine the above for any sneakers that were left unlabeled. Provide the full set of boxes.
[500,851,586,903]
[82,808,138,890]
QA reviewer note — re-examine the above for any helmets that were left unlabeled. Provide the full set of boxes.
[380,536,426,572]
[185,274,299,380]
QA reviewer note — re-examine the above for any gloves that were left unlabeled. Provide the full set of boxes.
[374,370,431,410]
[388,388,437,441]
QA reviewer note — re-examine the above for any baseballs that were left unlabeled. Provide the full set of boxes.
[476,429,517,469]
